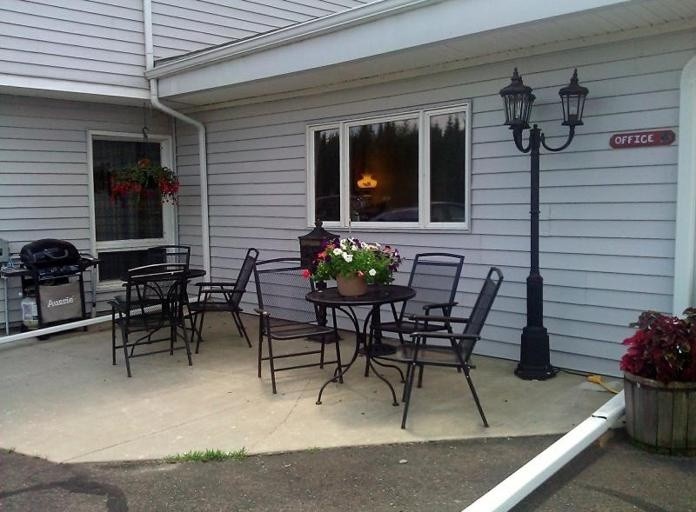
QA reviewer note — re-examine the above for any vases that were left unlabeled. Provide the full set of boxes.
[335,273,372,295]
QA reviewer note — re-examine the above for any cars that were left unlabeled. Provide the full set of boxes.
[369,199,465,223]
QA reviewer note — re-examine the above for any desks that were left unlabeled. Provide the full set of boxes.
[306,285,416,406]
[120,269,206,356]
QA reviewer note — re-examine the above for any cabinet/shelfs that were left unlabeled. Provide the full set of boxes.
[1,253,101,340]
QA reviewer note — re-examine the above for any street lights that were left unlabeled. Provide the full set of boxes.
[496,63,593,384]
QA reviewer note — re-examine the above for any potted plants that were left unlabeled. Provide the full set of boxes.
[620,307,696,452]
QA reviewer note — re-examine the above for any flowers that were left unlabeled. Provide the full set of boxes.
[302,237,400,289]
[107,158,181,211]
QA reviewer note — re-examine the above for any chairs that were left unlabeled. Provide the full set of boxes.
[107,263,192,377]
[187,248,258,355]
[396,267,504,430]
[366,253,464,388]
[253,258,344,394]
[140,245,202,345]
